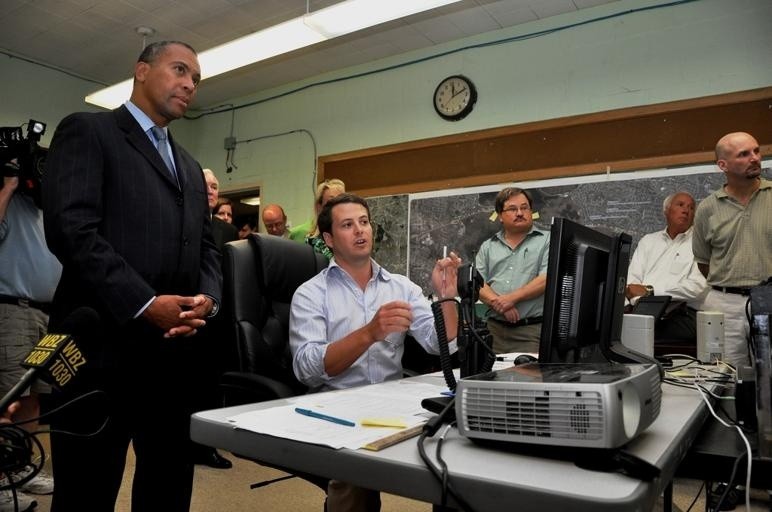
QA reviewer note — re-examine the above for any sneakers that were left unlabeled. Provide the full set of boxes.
[0,467,55,496]
[0,476,39,512]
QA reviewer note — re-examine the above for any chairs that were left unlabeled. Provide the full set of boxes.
[220,222,425,510]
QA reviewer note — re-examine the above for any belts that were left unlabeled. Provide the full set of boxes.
[711,282,772,297]
[0,293,55,316]
[485,314,545,329]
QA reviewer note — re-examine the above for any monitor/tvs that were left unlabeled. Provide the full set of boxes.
[538,216,632,383]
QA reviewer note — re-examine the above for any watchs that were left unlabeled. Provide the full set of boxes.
[644,285,653,297]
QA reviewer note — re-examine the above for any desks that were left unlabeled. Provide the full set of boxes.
[184,348,736,510]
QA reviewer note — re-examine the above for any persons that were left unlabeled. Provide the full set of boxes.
[623,191,709,355]
[476,187,551,354]
[193,169,347,468]
[691,132,772,456]
[288,193,464,395]
[1,41,224,511]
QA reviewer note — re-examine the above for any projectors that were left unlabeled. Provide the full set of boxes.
[454,362,662,449]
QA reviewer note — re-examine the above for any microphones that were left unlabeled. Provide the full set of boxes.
[0,305,109,419]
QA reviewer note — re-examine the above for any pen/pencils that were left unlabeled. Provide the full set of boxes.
[295,407,355,428]
[441,246,447,298]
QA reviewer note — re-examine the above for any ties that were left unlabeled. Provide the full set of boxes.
[149,125,181,188]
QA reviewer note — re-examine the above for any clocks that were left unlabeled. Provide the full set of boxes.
[430,71,478,124]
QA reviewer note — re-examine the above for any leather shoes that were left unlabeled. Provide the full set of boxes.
[193,444,233,470]
